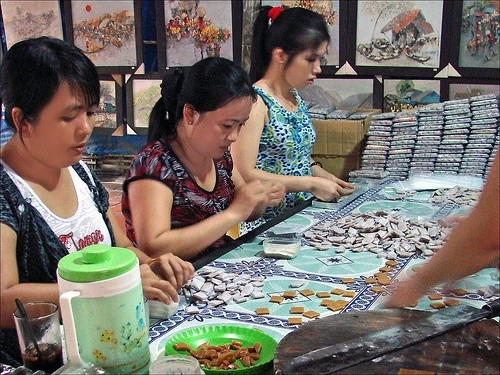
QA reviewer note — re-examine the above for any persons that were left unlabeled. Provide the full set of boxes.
[0,36,194,375]
[387,145,500,308]
[229,5,355,237]
[122,58,285,263]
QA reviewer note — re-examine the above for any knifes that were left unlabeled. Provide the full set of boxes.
[274,297,500,375]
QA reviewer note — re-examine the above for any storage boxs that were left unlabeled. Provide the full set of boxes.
[309,108,382,181]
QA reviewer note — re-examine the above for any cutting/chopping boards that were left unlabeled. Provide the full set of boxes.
[274,308,500,375]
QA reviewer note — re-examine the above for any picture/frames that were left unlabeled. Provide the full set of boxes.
[346,0,453,78]
[92,74,127,137]
[296,75,500,113]
[448,0,500,78]
[63,0,145,74]
[260,0,349,76]
[155,0,242,77]
[123,72,169,135]
[0,0,64,51]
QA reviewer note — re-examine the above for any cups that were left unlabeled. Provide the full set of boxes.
[143,295,149,341]
[148,354,207,375]
[12,301,63,375]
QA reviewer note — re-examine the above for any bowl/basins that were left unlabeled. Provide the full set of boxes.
[263,236,301,259]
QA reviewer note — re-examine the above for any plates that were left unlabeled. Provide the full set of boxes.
[165,324,277,374]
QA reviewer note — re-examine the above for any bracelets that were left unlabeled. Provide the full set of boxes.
[311,162,322,168]
[148,258,157,265]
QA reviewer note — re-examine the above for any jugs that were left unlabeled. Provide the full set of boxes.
[55,243,150,375]
